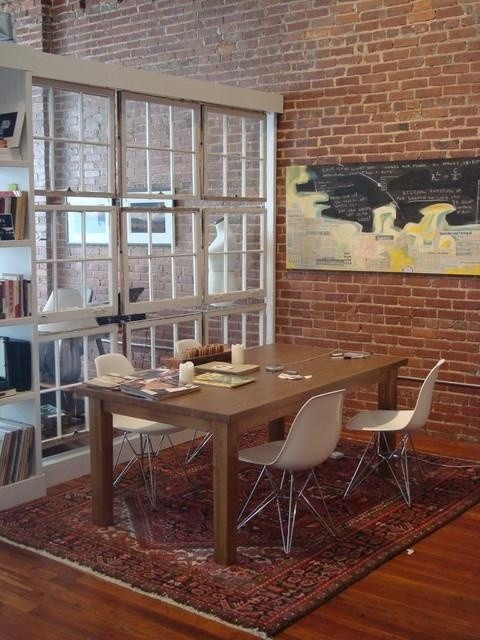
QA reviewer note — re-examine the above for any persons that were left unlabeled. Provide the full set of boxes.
[207,217,238,308]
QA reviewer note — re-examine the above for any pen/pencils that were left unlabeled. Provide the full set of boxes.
[344,356,365,359]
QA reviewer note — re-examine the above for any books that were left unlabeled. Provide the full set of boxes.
[0,102,35,488]
[84,359,262,401]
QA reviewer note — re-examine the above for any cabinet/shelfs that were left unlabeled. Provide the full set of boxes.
[0,43,47,512]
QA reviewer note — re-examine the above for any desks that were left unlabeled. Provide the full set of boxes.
[76,343,408,567]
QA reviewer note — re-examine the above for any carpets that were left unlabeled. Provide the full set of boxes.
[0,427,480,640]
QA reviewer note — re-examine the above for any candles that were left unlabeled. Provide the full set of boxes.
[231,344,244,364]
[179,360,195,383]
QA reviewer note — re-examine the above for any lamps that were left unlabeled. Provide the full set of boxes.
[38,286,99,358]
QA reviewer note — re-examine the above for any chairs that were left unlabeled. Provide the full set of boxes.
[237,387,346,558]
[342,359,444,508]
[174,338,213,465]
[94,353,190,511]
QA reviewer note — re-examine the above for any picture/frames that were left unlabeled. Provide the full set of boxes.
[0,111,26,148]
[65,197,110,246]
[127,189,176,246]
